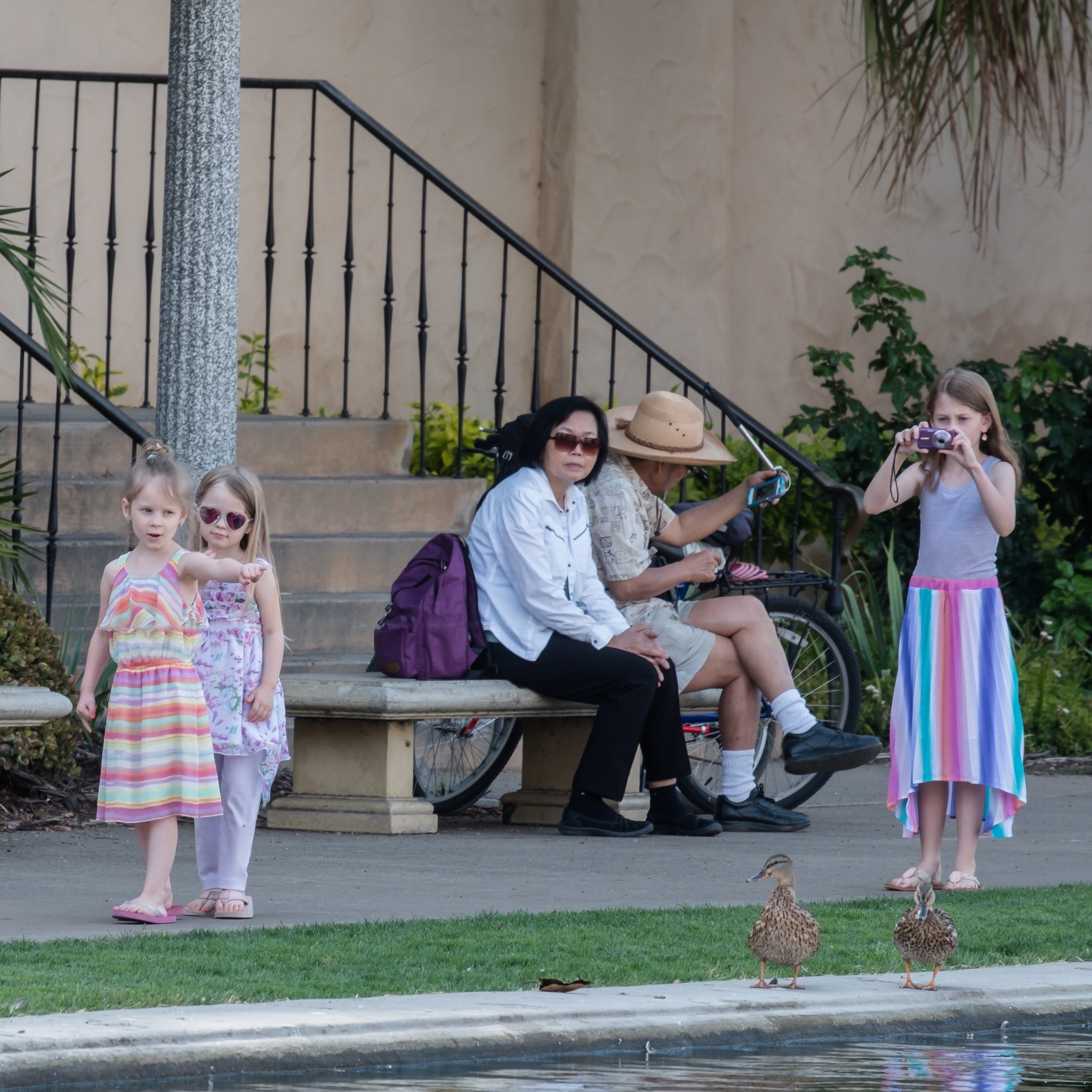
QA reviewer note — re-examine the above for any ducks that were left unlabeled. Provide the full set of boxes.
[893,882,959,992]
[746,854,821,989]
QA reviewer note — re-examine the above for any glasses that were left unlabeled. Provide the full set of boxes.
[548,432,602,457]
[197,503,255,532]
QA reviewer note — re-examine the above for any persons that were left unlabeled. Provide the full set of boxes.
[462,394,725,839]
[585,383,884,833]
[73,436,272,924]
[861,366,1028,895]
[178,463,295,917]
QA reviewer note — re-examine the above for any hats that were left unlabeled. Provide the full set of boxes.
[604,390,738,464]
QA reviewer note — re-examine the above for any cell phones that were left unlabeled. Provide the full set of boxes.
[747,477,788,507]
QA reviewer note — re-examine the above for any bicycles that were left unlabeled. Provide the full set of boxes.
[406,501,865,818]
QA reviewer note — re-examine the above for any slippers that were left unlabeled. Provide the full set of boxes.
[112,899,176,924]
[166,905,184,916]
[214,890,254,918]
[184,891,218,916]
[945,871,983,891]
[885,867,944,891]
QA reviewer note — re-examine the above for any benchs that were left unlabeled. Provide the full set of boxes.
[258,671,728,835]
[0,684,75,729]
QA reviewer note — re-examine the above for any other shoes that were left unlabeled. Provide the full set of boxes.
[646,809,722,836]
[558,806,654,837]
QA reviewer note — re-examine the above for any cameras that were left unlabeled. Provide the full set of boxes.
[917,427,955,450]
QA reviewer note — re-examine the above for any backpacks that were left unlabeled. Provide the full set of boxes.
[369,533,500,680]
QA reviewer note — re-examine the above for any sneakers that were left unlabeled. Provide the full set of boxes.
[780,722,883,775]
[712,784,811,833]
